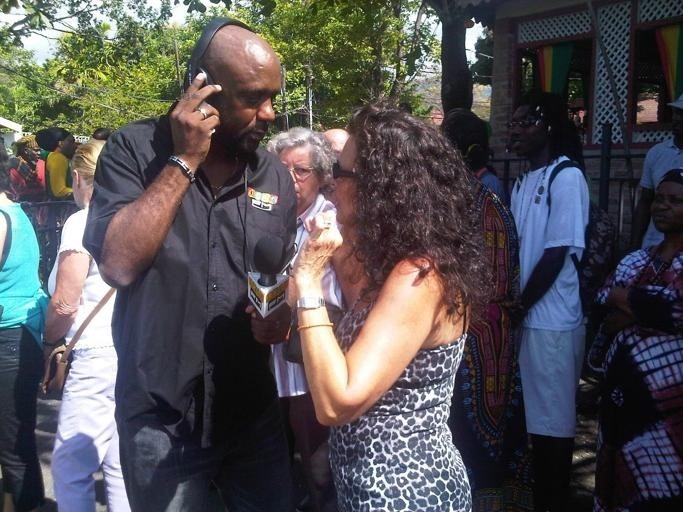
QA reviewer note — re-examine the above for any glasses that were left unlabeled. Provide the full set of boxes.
[507,114,538,128]
[287,164,315,182]
[332,160,356,179]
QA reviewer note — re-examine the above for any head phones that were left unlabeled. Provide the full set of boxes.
[184,17,256,100]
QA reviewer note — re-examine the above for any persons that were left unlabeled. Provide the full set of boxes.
[81,17,297,509]
[264,92,683,512]
[0,127,132,512]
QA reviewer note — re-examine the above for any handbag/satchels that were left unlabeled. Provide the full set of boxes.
[39,338,74,397]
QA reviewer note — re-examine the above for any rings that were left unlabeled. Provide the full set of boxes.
[196,106,207,119]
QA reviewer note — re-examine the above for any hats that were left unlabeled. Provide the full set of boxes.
[667,94,683,109]
[17,135,41,150]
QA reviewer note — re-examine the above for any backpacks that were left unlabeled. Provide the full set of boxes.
[517,160,615,317]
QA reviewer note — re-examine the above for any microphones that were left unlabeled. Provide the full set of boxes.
[253,235,284,318]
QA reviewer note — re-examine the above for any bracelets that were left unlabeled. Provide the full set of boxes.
[168,156,195,183]
